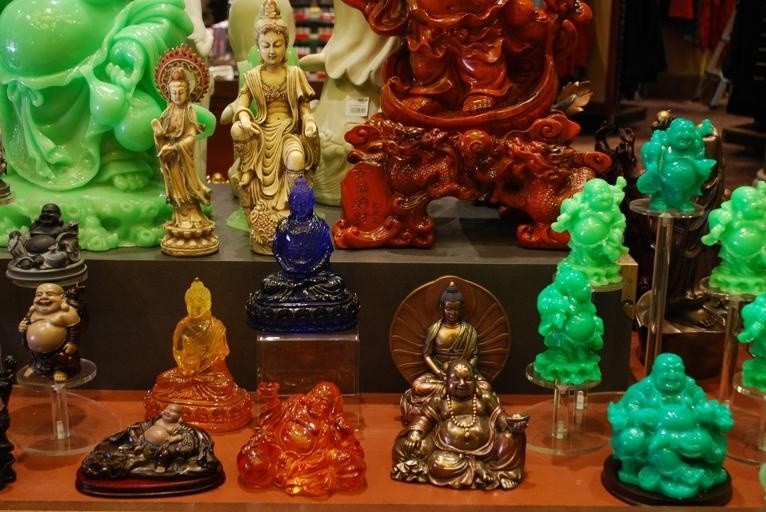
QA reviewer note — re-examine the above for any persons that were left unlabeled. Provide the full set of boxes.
[356,1,766,508]
[1,1,366,501]
